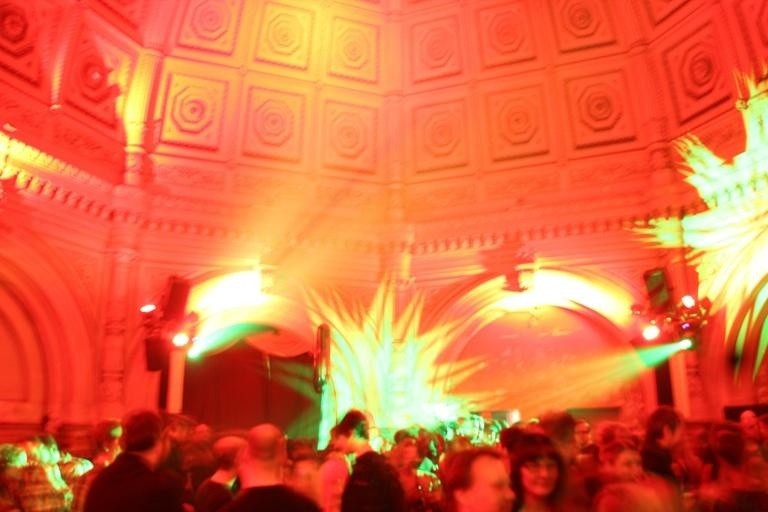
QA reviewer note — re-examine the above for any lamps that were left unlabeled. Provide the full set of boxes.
[137,272,200,410]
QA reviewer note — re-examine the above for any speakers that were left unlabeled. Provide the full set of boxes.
[144,335,164,371]
[643,266,673,313]
